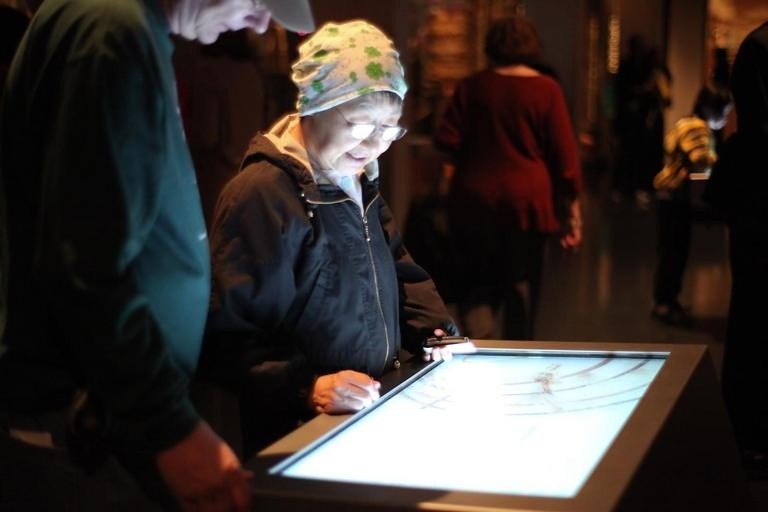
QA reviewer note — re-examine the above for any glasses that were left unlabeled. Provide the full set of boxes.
[334,105,409,143]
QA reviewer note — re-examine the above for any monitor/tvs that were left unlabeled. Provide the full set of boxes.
[249,338,710,512]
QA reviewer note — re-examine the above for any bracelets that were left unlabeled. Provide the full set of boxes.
[566,217,583,231]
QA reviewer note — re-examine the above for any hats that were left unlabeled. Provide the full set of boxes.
[256,0,315,35]
[291,19,409,118]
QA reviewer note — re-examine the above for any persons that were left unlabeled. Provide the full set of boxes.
[602,16,768,484]
[433,18,585,341]
[163,24,283,460]
[207,18,461,457]
[2,0,317,512]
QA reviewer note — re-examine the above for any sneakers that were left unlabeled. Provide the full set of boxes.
[650,305,693,328]
